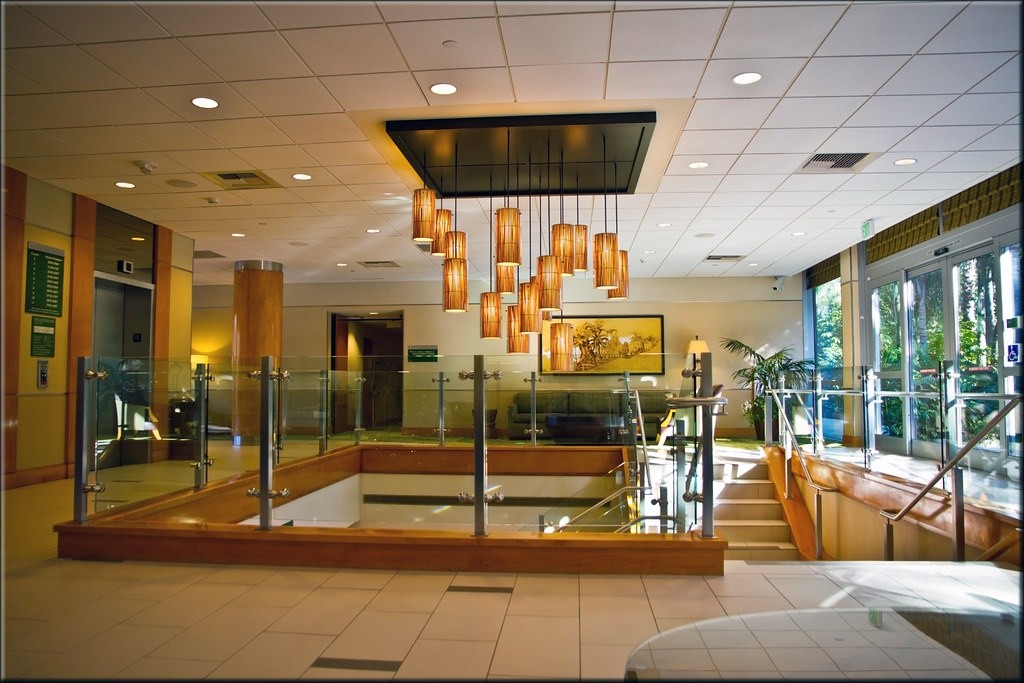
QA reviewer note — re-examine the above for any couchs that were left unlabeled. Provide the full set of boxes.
[504,389,695,442]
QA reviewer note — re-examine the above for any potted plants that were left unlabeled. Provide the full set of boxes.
[718,335,822,440]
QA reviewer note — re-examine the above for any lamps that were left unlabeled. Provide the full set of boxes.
[687,335,710,363]
[380,109,658,372]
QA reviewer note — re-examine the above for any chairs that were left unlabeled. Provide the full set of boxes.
[674,384,725,438]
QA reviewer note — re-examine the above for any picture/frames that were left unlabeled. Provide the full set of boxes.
[538,315,665,375]
